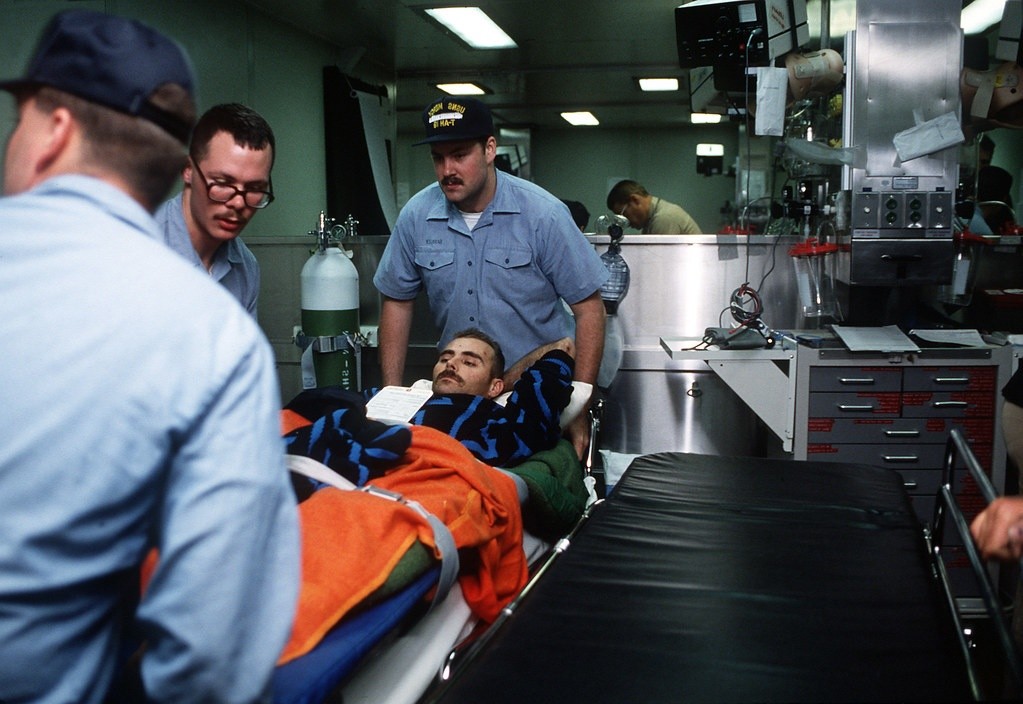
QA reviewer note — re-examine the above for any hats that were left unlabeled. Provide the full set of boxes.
[412,95,494,147]
[0,8,196,143]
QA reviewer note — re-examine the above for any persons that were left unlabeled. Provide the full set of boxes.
[607,179,703,236]
[972,358,1023,560]
[149,100,276,325]
[371,96,612,468]
[283,330,578,510]
[559,199,592,233]
[0,10,302,704]
[965,135,1017,236]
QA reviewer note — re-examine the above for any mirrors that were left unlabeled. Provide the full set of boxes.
[0,0,1023,248]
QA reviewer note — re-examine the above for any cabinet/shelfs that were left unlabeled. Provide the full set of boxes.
[791,349,1004,600]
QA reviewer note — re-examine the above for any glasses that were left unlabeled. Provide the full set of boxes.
[189,152,276,210]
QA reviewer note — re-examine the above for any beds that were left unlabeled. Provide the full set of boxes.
[415,427,1023,704]
[266,446,637,704]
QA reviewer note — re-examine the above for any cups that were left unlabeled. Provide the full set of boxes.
[789,238,837,319]
[938,230,981,308]
